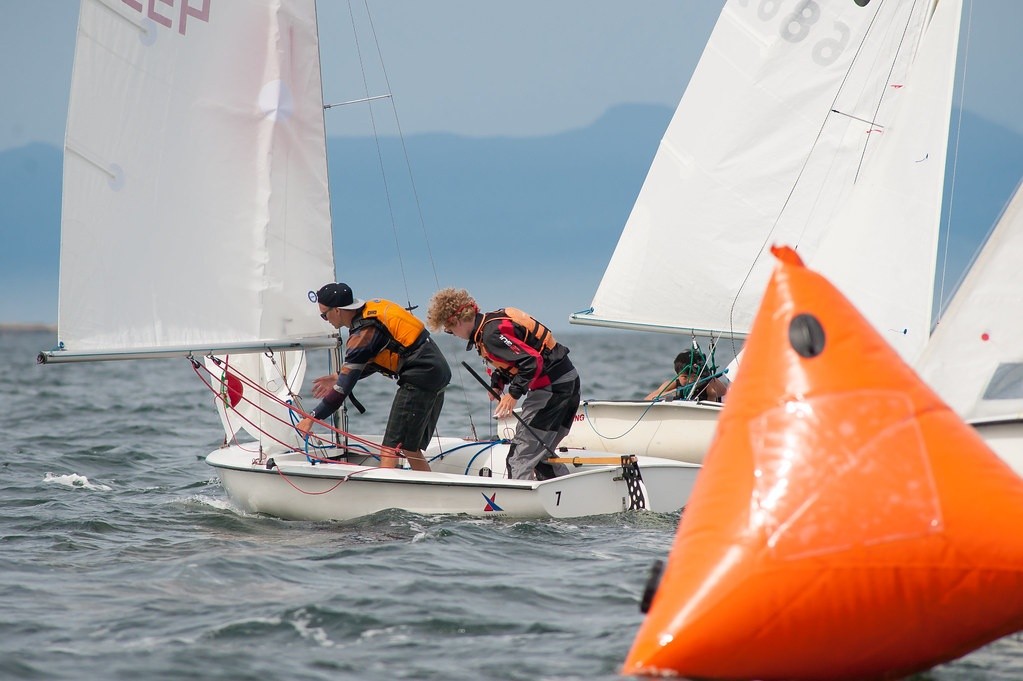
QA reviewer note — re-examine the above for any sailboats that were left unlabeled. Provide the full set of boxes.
[34,0,707,524]
[492,0,1023,480]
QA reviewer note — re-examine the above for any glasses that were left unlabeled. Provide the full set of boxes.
[444,322,453,334]
[320,307,332,320]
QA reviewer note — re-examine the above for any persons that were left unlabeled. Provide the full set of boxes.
[296,282,452,472]
[427,288,581,482]
[707,376,731,401]
[644,350,722,403]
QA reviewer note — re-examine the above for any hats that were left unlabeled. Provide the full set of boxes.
[317,282,367,310]
